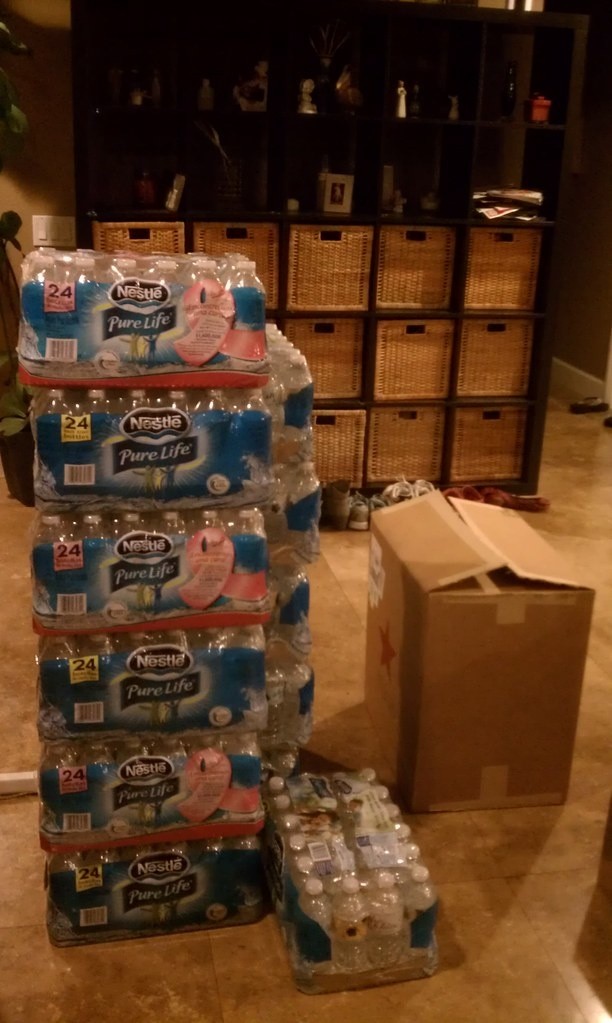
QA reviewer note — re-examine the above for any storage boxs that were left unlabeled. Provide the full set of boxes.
[369,488,597,812]
[90,217,543,490]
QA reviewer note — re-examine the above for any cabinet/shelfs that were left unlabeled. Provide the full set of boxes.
[70,0,590,494]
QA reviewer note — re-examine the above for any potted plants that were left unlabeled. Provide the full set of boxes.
[1,21,37,508]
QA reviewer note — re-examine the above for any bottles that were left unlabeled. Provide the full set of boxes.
[15,247,440,995]
